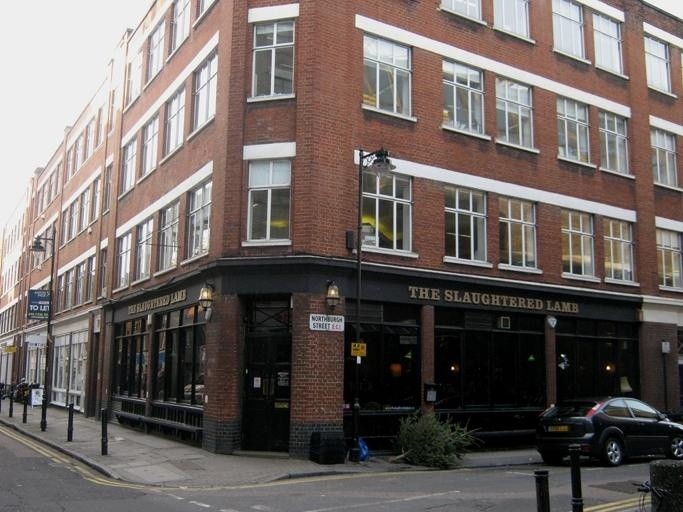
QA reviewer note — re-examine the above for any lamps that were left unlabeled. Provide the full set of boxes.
[324,283,339,308]
[196,283,212,310]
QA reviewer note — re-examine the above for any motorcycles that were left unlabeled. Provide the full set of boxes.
[12,381,39,407]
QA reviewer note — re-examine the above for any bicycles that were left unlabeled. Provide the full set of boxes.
[632,478,682,512]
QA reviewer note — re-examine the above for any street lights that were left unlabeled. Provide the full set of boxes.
[29,226,56,435]
[352,144,396,461]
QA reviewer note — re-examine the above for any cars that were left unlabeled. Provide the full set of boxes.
[536,397,683,467]
[183,372,205,404]
